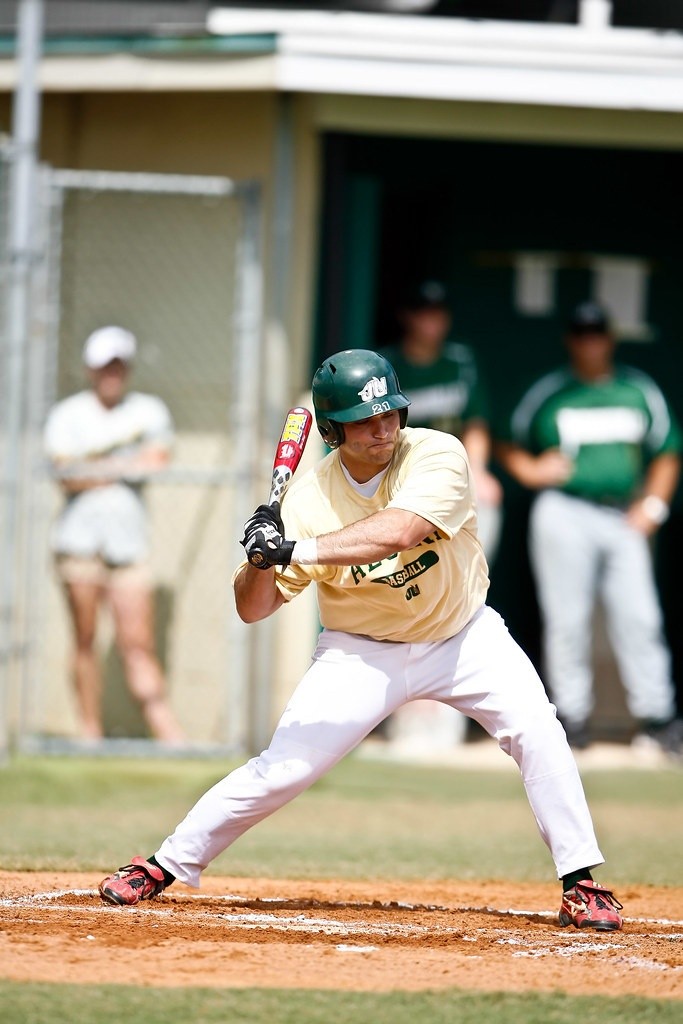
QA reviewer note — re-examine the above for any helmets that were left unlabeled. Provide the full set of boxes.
[311,348,412,449]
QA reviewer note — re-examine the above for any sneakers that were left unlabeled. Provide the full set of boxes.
[633,715,683,754]
[98,854,176,906]
[564,719,594,748]
[559,879,624,931]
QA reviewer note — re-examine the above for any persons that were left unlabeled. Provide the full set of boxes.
[98,347,623,933]
[377,280,504,568]
[42,324,186,742]
[494,300,683,752]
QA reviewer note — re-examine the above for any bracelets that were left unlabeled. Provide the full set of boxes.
[634,488,668,525]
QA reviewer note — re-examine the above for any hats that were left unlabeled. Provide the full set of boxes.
[81,326,138,370]
[564,298,610,338]
[403,276,451,313]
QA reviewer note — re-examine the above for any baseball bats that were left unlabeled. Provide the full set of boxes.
[246,406,312,569]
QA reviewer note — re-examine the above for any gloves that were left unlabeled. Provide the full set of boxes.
[245,522,296,570]
[239,501,285,570]
